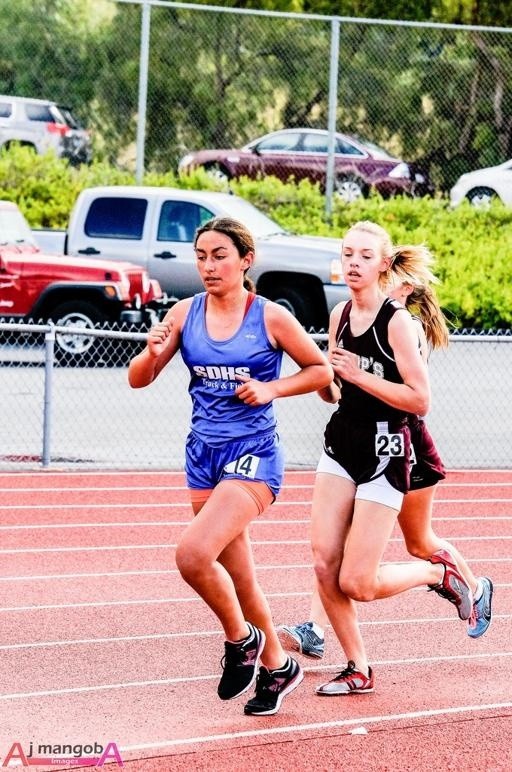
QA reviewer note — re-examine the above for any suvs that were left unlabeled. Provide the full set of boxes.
[0,199,180,367]
[0,94,90,169]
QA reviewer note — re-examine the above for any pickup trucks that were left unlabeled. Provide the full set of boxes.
[31,186,351,316]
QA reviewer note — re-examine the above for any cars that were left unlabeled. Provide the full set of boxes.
[448,159,512,208]
[177,128,415,201]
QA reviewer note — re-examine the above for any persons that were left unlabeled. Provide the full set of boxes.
[311,219,474,697]
[129,217,334,718]
[278,275,493,660]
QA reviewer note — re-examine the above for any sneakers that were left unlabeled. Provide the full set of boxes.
[244,654,305,718]
[426,549,475,621]
[275,619,325,661]
[467,576,494,639]
[216,621,267,701]
[313,661,375,695]
